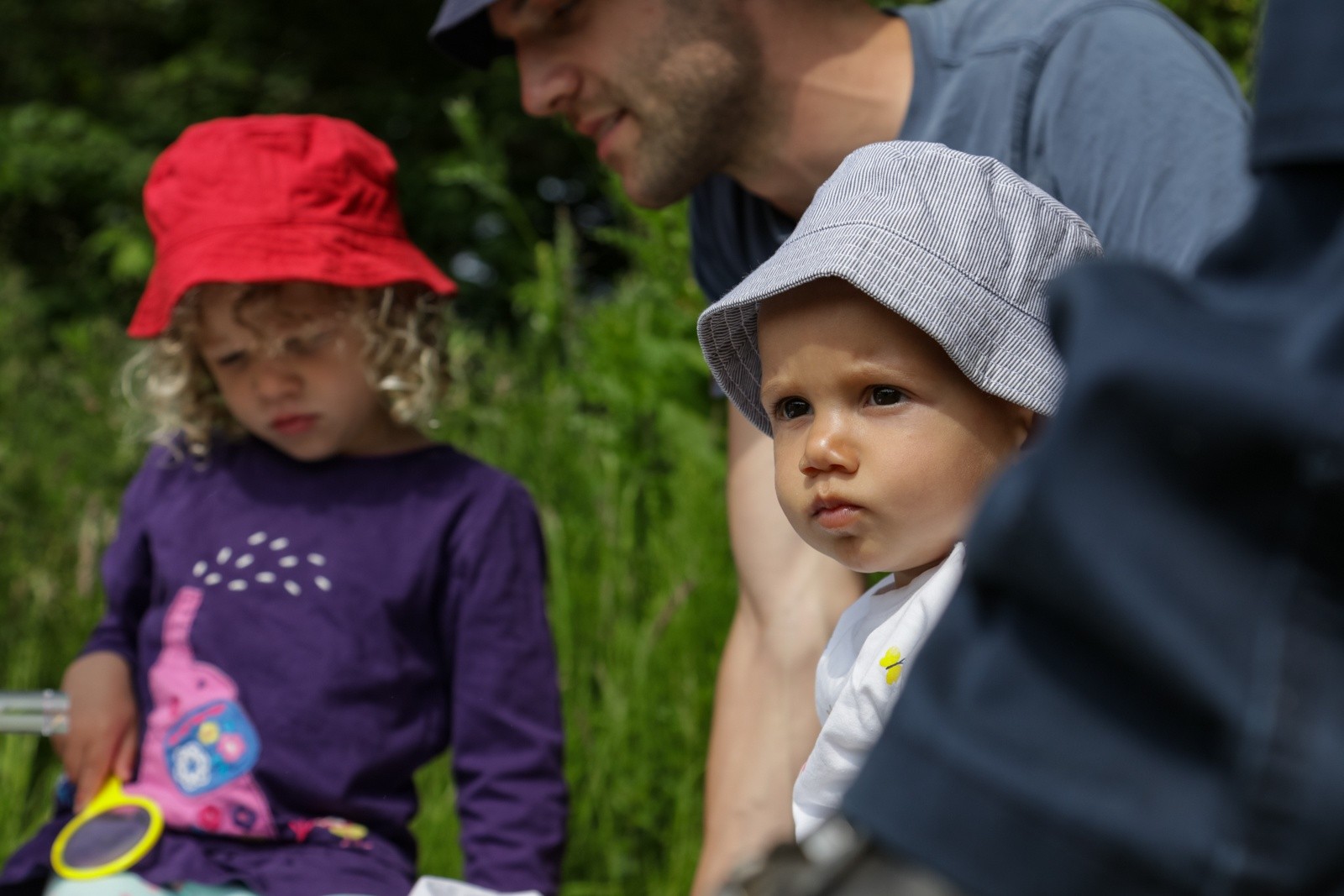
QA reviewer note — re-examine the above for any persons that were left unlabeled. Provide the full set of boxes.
[0,112,570,896]
[428,0,1257,896]
[707,0,1344,895]
[696,140,1107,847]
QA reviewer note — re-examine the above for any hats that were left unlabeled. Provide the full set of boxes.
[428,0,491,36]
[698,141,1105,437]
[126,114,461,341]
